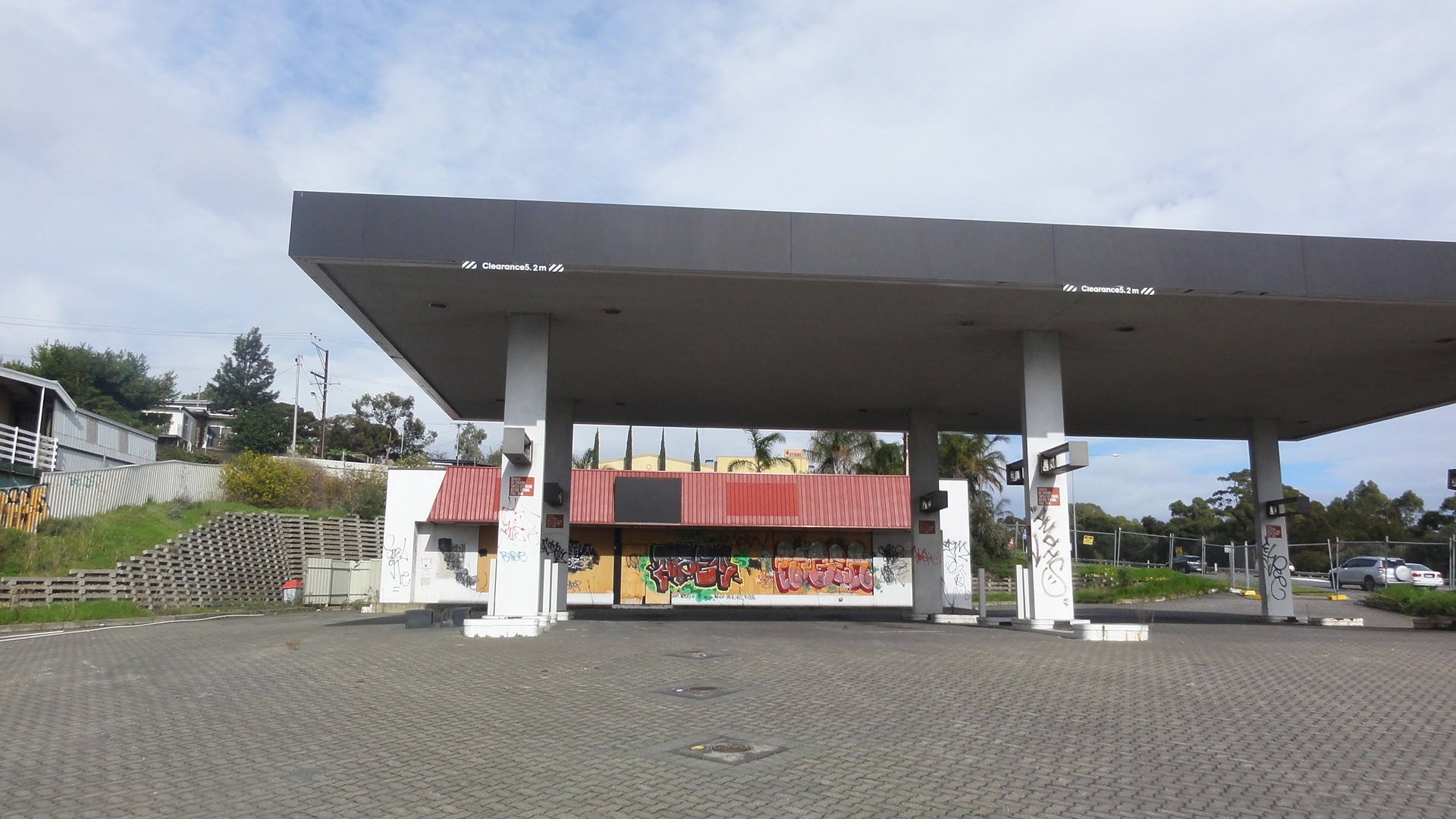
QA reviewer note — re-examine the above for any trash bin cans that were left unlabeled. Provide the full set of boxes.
[282,579,302,606]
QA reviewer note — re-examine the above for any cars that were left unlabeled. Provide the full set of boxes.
[1165,555,1208,574]
[1405,563,1444,590]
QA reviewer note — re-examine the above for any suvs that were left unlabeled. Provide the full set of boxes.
[1328,555,1414,591]
